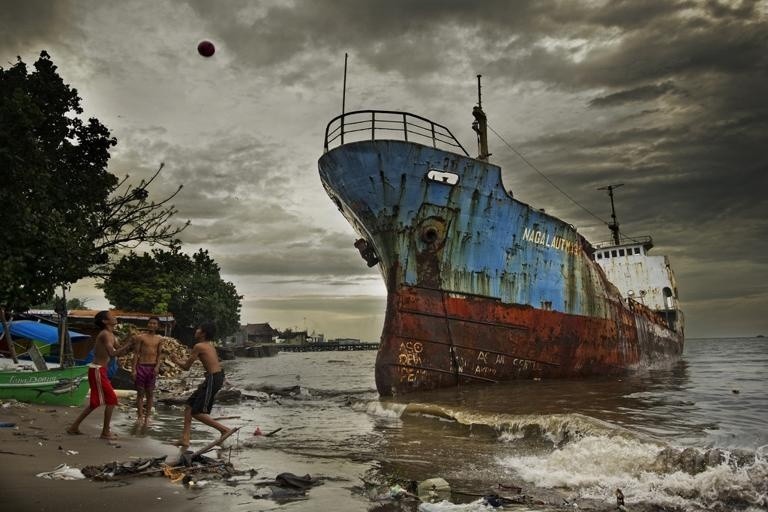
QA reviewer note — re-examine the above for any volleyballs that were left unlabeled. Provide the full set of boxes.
[198,41,214,57]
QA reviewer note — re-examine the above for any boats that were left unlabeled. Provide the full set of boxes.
[0,319,91,370]
[0,365,90,407]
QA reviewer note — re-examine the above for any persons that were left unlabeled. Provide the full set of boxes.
[132,315,163,426]
[67,310,136,440]
[170,320,230,447]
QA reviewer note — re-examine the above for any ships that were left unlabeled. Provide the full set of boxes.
[317,51,686,396]
